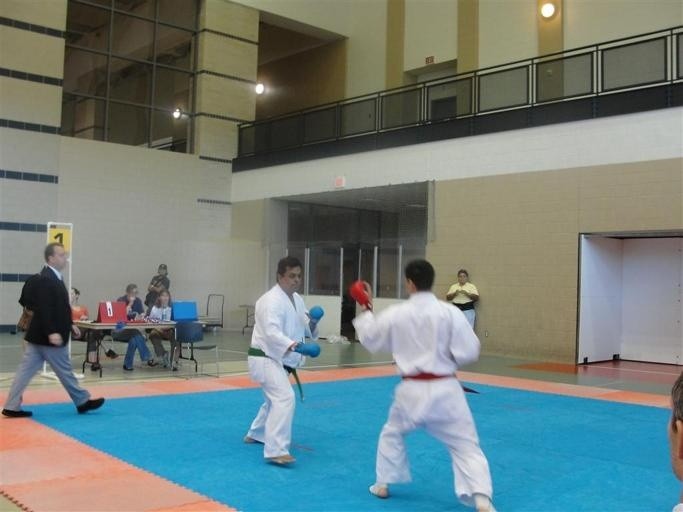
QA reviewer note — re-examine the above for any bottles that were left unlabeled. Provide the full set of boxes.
[161,305,166,320]
[128,307,133,315]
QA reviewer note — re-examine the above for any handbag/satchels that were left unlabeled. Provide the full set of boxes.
[144,292,152,306]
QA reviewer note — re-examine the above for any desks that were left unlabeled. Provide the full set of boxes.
[238,304,255,333]
[75,321,176,378]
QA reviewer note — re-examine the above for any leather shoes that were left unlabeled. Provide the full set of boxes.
[2,409,32,417]
[77,398,104,413]
[106,349,118,359]
[148,359,159,366]
[123,365,134,370]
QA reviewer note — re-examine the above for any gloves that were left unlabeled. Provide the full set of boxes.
[309,305,324,325]
[350,281,373,309]
[294,342,320,358]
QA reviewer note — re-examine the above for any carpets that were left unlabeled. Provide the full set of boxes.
[0,363,683,511]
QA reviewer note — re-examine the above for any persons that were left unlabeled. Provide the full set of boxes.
[447,269,479,330]
[147,290,172,368]
[1,243,105,418]
[243,257,324,465]
[668,372,683,512]
[71,287,118,371]
[349,259,498,512]
[144,263,170,318]
[116,284,158,370]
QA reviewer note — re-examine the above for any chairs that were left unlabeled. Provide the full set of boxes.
[178,320,220,377]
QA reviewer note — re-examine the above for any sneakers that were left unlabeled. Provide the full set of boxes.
[163,350,169,367]
